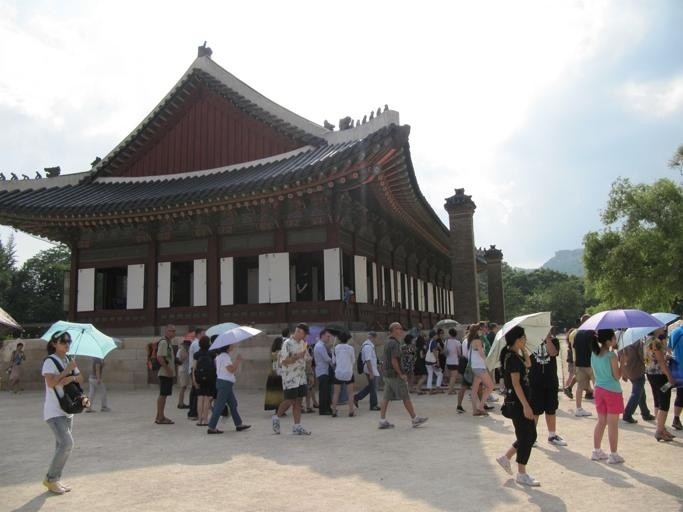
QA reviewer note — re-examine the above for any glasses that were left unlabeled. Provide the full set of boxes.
[58,339,71,344]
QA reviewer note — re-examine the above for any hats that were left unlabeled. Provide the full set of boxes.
[298,324,310,335]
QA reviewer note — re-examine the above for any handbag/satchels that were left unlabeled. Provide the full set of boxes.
[463,366,473,383]
[425,351,437,363]
[500,396,516,418]
[460,356,468,366]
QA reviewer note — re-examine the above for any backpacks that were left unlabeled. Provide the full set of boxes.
[44,354,91,413]
[357,344,370,374]
[196,350,216,382]
[146,339,170,370]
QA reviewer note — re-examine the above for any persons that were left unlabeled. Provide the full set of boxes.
[85,358,111,412]
[296,271,310,301]
[265,322,382,435]
[43,332,91,494]
[529,331,567,447]
[342,286,353,331]
[399,321,502,415]
[8,343,26,393]
[379,322,428,429]
[156,322,252,434]
[496,326,539,486]
[567,314,682,464]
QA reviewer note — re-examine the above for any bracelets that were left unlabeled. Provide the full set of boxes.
[72,376,75,382]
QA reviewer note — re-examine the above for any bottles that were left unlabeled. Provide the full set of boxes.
[660,382,675,393]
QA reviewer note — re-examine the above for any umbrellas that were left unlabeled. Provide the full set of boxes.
[487,311,552,371]
[577,310,665,354]
[0,308,26,333]
[42,320,117,360]
[618,313,679,352]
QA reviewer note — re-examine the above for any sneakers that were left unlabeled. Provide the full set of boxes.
[548,435,567,446]
[379,421,394,429]
[413,416,427,428]
[102,407,110,411]
[43,478,71,493]
[301,396,358,416]
[155,416,174,424]
[293,425,311,435]
[85,407,96,412]
[585,391,594,400]
[272,414,281,434]
[188,411,208,426]
[456,393,498,416]
[591,449,624,463]
[207,430,224,434]
[368,406,380,410]
[516,473,540,486]
[177,404,190,409]
[622,411,683,442]
[575,408,592,417]
[236,424,250,431]
[497,454,514,475]
[564,384,573,398]
[409,384,456,395]
